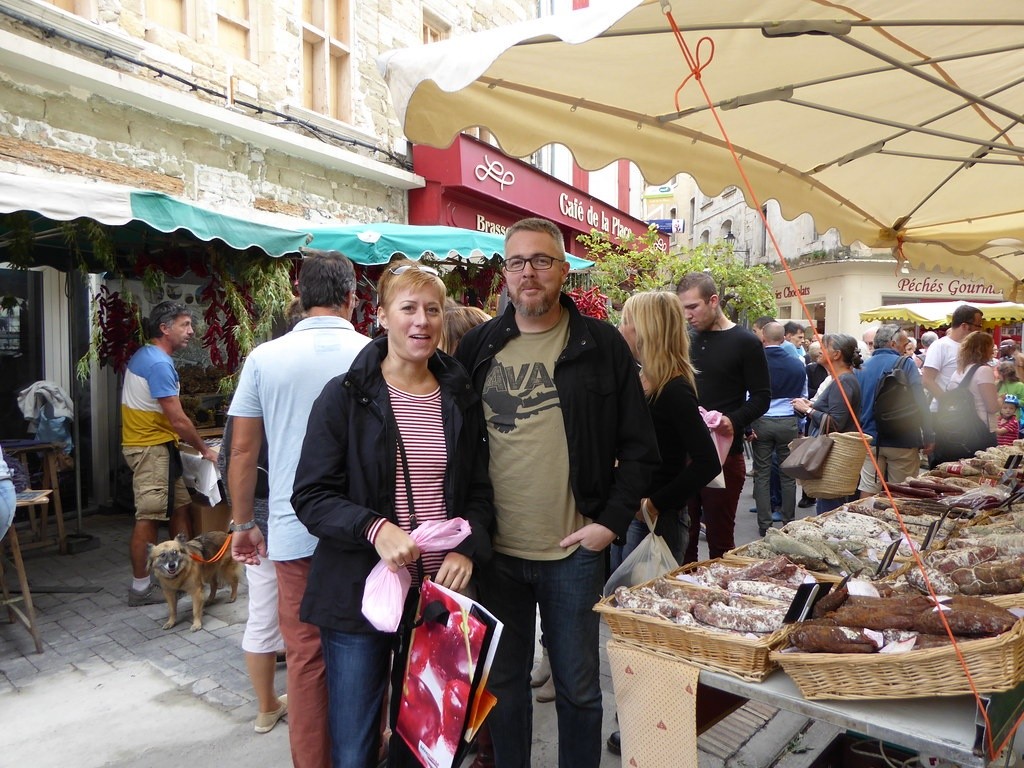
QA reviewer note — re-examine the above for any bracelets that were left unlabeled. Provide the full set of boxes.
[229,519,255,531]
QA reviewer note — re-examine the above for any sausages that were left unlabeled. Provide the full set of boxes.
[613,480,1024,654]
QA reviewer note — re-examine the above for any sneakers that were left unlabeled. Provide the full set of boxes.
[129,585,180,606]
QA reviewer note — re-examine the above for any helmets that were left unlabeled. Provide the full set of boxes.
[1003,395,1019,408]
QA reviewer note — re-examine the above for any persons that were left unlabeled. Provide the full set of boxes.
[120,301,221,607]
[224,297,314,732]
[290,259,495,768]
[530,621,560,704]
[606,291,719,758]
[227,251,375,768]
[675,271,771,571]
[743,303,1024,537]
[431,296,500,358]
[0,444,17,541]
[453,219,663,768]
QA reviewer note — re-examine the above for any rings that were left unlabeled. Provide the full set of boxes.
[397,561,406,566]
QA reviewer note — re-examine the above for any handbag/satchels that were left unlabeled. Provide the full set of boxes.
[604,499,679,596]
[777,413,873,499]
[697,405,734,489]
[363,514,505,768]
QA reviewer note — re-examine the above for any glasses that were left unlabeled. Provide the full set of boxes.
[890,326,902,341]
[500,254,564,273]
[960,321,983,331]
[381,264,440,300]
[157,303,189,321]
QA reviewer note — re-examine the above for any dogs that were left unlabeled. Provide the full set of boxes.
[145,530,244,633]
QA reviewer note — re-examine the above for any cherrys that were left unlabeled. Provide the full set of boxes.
[395,582,488,757]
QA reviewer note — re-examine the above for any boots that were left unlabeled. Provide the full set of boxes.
[535,678,555,702]
[530,640,552,687]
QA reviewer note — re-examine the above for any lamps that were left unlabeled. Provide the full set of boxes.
[724,230,749,253]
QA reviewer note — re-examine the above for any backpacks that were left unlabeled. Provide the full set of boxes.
[872,355,923,438]
[931,360,993,457]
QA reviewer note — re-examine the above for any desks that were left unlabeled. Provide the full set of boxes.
[607,638,1024,768]
[0,495,53,653]
[0,438,66,554]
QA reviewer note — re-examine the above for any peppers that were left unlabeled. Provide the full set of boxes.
[444,264,506,301]
[565,285,609,322]
[93,259,382,378]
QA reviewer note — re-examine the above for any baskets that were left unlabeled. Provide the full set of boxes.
[593,466,1023,700]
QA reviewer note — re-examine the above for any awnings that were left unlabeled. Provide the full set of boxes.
[0,158,600,276]
[859,299,1024,330]
[377,0,1024,304]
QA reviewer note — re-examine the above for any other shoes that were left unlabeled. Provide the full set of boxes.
[699,521,707,535]
[798,494,818,508]
[606,730,621,754]
[770,509,783,521]
[276,647,286,662]
[255,693,289,731]
[468,744,495,768]
[749,508,758,513]
